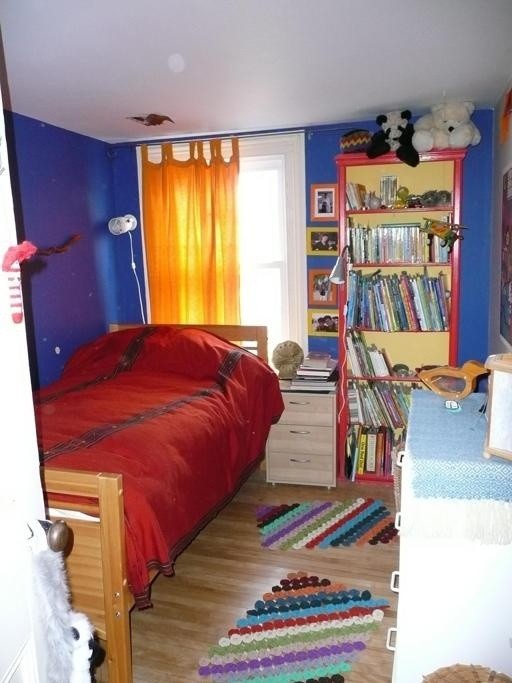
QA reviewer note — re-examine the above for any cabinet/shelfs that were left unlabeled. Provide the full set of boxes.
[378,386,511,682]
[334,149,467,487]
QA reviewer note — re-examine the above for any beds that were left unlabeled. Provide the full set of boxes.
[34,318,276,681]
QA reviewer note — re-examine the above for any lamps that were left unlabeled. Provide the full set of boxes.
[109,213,138,238]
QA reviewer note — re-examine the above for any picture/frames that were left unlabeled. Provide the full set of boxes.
[308,181,338,222]
[306,227,343,257]
[305,267,338,307]
[306,306,341,339]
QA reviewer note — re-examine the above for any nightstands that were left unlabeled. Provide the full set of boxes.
[264,360,342,491]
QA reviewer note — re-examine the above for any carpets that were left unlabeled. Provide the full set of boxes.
[250,496,402,553]
[198,569,388,682]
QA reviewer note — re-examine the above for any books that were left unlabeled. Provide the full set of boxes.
[344,174,454,482]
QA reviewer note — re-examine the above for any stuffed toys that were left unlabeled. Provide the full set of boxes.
[365,109,420,167]
[412,100,482,152]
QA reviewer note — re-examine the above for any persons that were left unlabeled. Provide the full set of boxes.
[312,193,338,334]
